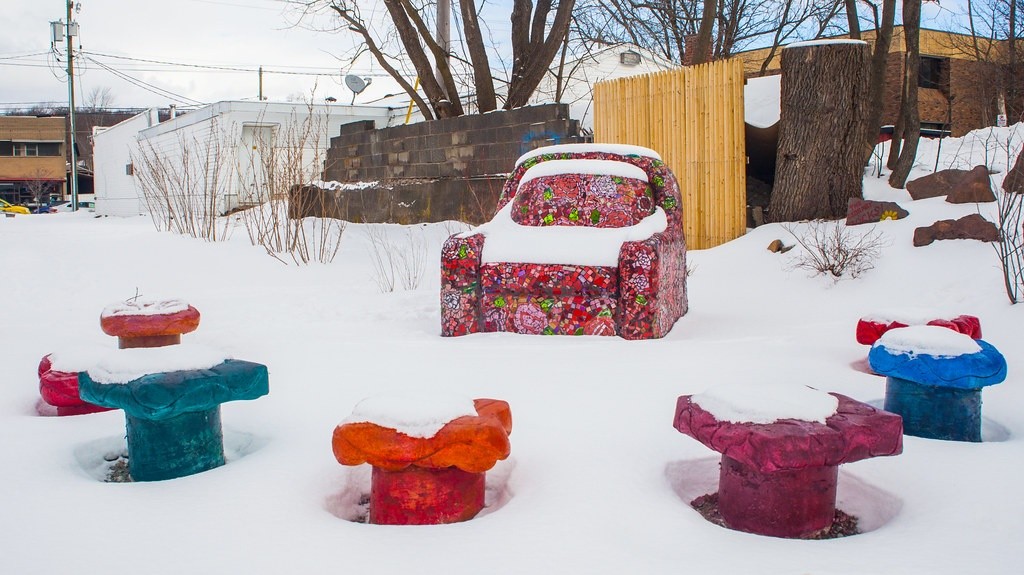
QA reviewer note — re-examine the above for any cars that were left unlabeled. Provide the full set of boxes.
[33,200,95,214]
[0,198,31,214]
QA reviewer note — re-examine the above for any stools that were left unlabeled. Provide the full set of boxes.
[857,316,982,377]
[99,297,201,348]
[674,391,902,539]
[869,338,1006,442]
[332,399,513,525]
[77,360,268,483]
[38,352,120,416]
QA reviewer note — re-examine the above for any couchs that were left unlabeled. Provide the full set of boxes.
[440,143,689,340]
